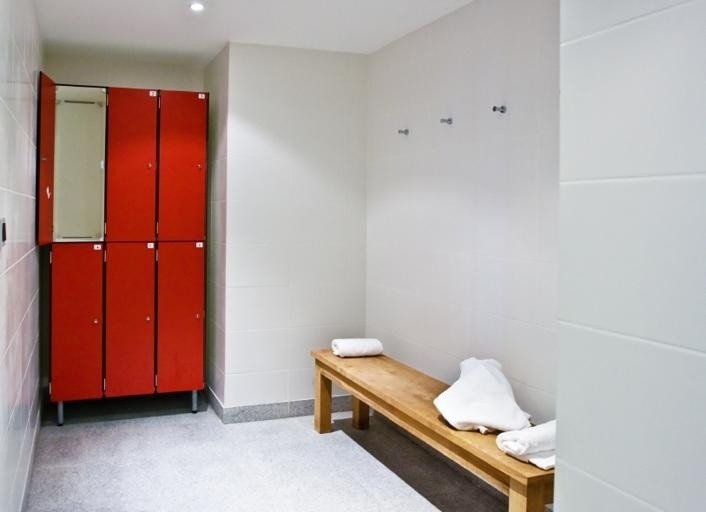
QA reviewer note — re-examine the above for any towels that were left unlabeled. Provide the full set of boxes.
[331,338,383,357]
[432,356,533,434]
[496,419,555,470]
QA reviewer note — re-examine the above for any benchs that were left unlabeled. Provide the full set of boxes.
[311,345,554,512]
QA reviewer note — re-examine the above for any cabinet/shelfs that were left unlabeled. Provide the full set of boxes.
[36,71,210,426]
